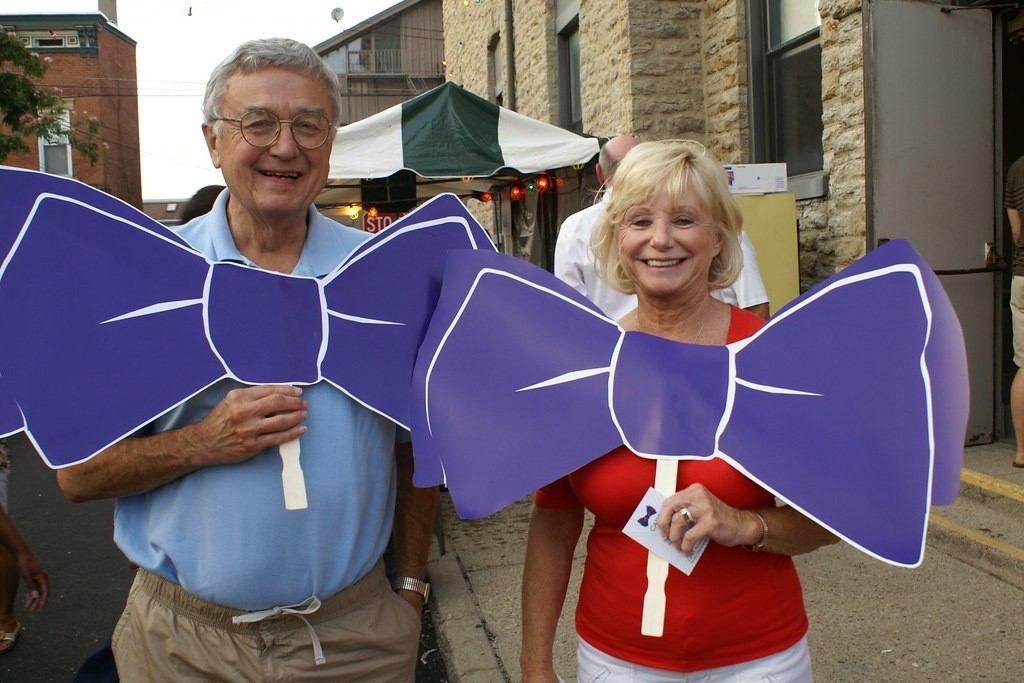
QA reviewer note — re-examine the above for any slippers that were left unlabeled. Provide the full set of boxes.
[0,622,20,653]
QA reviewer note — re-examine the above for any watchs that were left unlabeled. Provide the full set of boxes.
[393,578,431,604]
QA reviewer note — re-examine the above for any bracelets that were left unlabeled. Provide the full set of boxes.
[742,508,769,553]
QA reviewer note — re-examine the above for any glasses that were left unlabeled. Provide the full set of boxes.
[210,109,337,149]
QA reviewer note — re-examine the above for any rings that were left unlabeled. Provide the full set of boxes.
[681,509,694,525]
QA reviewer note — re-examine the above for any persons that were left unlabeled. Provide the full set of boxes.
[1005,154,1024,467]
[0,36,439,683]
[553,134,770,330]
[520,137,840,683]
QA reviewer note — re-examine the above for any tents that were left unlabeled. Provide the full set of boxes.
[308,81,609,262]
